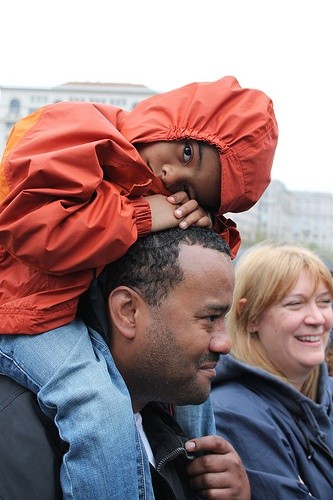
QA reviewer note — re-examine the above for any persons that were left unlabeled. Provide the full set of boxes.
[1,227,250,500]
[1,75,279,500]
[213,239,333,500]
